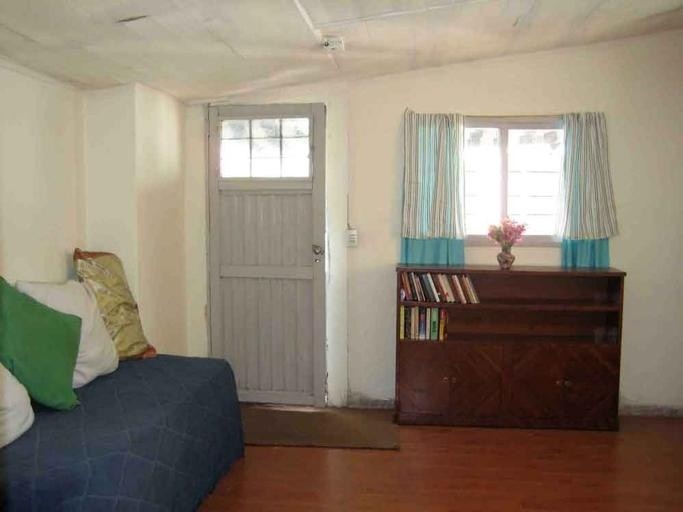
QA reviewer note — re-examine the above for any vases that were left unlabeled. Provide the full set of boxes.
[494,240,516,271]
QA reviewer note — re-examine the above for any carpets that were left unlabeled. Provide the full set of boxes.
[237,404,402,452]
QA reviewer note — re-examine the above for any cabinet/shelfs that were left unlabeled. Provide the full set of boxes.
[506,341,622,430]
[394,340,506,427]
[390,261,628,343]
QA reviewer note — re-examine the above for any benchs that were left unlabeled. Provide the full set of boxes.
[1,354,248,511]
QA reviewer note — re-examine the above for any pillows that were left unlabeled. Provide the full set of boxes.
[72,247,158,359]
[0,277,83,412]
[1,362,35,450]
[17,275,120,393]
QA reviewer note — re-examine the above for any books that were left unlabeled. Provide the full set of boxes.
[398,270,480,343]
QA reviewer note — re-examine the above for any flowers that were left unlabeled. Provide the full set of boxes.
[484,215,526,249]
